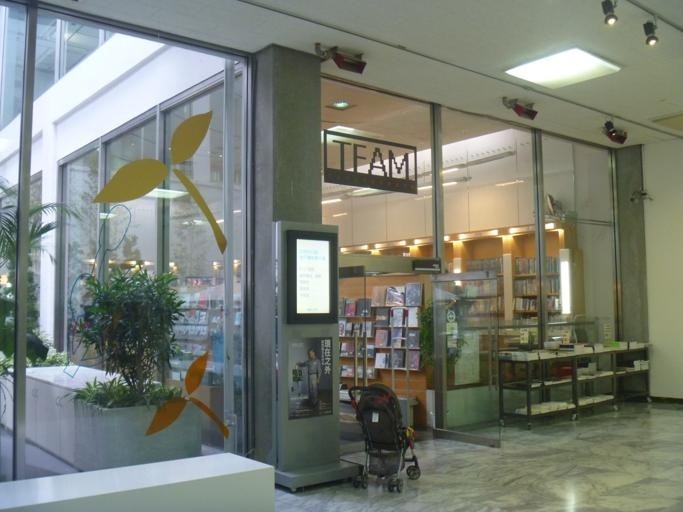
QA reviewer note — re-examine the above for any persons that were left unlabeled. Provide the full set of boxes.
[295,347,323,407]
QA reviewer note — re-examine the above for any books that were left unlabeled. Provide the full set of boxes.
[176,327,210,340]
[371,282,423,307]
[520,378,562,387]
[338,296,375,317]
[466,256,503,274]
[339,319,375,338]
[340,341,374,356]
[513,297,561,313]
[602,360,648,376]
[516,401,576,416]
[540,340,594,360]
[467,297,503,314]
[374,328,421,348]
[464,280,503,299]
[175,311,223,325]
[499,341,540,362]
[373,308,421,327]
[560,361,599,380]
[595,339,653,352]
[339,383,355,402]
[514,278,559,296]
[574,393,615,406]
[341,365,378,379]
[515,254,557,275]
[179,291,223,311]
[375,350,420,370]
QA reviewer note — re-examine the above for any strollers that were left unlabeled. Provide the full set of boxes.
[348,383,420,492]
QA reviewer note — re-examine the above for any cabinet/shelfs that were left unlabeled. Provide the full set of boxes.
[597,347,651,410]
[545,350,614,420]
[339,275,432,428]
[498,355,575,429]
[452,258,560,349]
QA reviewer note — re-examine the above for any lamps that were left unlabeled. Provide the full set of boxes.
[600,0,618,26]
[642,19,658,48]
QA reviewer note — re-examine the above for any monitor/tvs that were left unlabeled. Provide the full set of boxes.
[287,229,337,324]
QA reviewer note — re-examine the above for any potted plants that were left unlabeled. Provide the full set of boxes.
[71,259,198,471]
